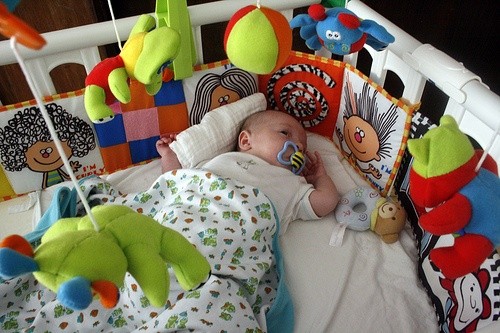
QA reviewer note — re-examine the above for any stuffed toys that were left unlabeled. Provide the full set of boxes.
[0,202,212,310]
[407,113,499,282]
[223,4,294,76]
[290,3,395,57]
[335,185,408,244]
[83,12,182,125]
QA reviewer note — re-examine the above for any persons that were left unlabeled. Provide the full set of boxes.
[155,109,341,237]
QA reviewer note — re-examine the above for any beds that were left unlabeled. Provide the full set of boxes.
[0,0,500,333]
